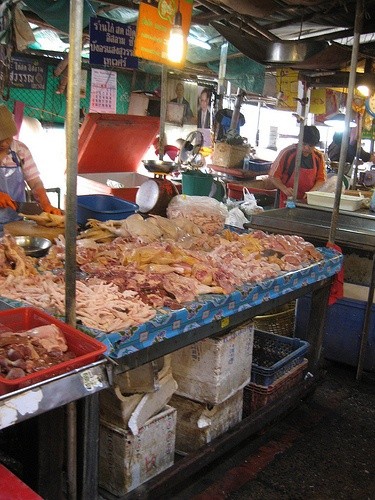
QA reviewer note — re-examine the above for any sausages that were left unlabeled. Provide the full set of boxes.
[53,56,86,98]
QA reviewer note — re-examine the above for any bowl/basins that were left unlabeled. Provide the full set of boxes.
[13,235,51,257]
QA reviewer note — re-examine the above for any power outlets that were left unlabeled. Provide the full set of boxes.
[324,113,358,128]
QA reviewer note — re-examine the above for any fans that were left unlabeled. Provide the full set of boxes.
[176,131,204,165]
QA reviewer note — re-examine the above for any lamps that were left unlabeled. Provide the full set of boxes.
[168,0,184,64]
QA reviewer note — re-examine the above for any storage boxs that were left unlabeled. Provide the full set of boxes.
[64,113,182,204]
[212,142,250,168]
[0,307,107,396]
[322,283,375,370]
[98,323,309,495]
[249,160,272,173]
[306,191,365,211]
[75,194,139,225]
[128,90,187,128]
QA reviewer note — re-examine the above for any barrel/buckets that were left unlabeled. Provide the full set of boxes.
[181,172,214,197]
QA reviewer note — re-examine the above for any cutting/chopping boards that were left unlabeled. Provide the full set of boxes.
[5,220,79,243]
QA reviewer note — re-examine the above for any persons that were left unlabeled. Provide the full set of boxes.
[327,132,371,171]
[0,106,63,237]
[170,82,195,121]
[197,89,211,129]
[269,125,325,207]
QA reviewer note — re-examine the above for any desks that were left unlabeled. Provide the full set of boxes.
[208,164,257,181]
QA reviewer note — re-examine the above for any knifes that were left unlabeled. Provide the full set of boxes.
[11,200,42,215]
